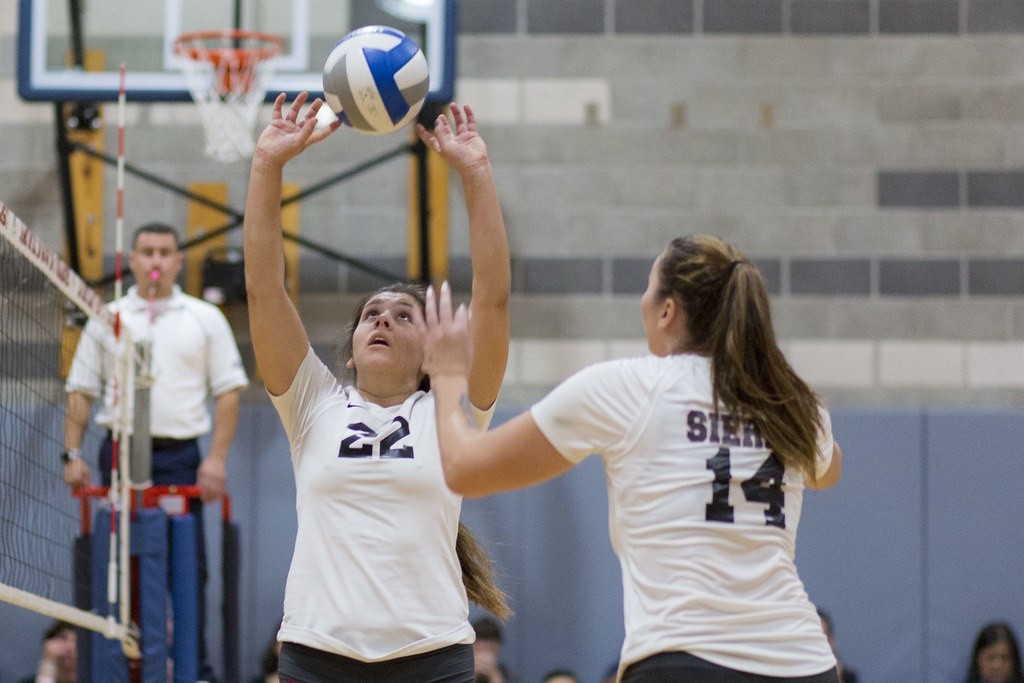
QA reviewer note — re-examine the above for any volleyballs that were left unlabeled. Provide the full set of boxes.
[320,22,431,139]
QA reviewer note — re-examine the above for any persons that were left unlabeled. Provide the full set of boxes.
[60,223,249,683]
[412,234,844,683]
[966,624,1023,683]
[470,617,508,683]
[243,92,512,683]
[20,621,77,683]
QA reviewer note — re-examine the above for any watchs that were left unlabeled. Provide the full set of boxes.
[59,448,76,461]
